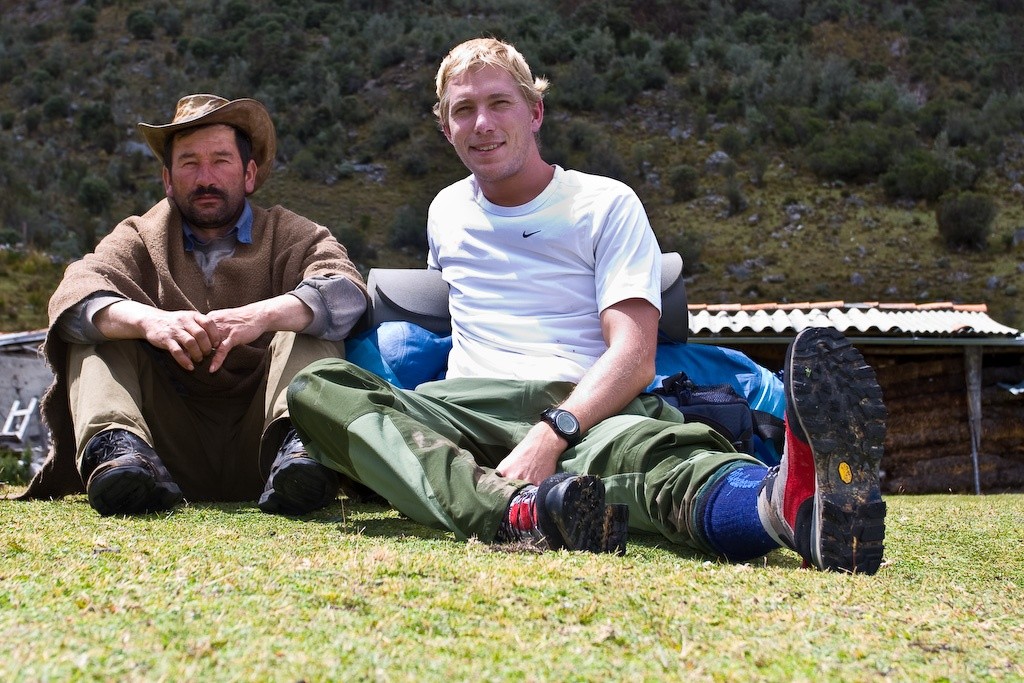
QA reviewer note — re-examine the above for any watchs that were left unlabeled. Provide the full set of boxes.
[541,405,583,447]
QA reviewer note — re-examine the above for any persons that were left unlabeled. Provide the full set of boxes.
[288,38,890,579]
[45,95,373,520]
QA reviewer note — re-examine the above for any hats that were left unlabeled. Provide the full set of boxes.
[138,92,275,195]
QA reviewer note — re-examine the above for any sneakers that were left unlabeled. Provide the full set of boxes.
[86,429,183,516]
[257,429,338,518]
[759,326,887,576]
[495,471,628,554]
